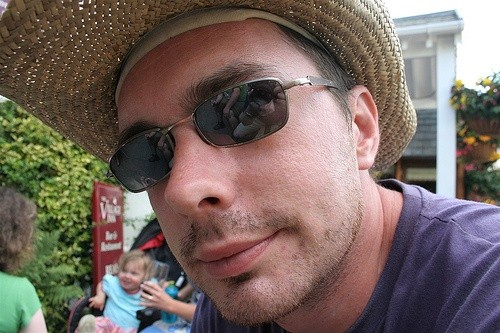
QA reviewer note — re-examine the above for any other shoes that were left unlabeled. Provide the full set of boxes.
[78,314,96,333]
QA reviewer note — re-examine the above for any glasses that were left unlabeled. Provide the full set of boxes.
[108,77,339,192]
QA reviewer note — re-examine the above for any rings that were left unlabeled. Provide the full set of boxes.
[147,295,153,301]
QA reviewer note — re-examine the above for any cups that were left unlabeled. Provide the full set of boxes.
[139,261,170,303]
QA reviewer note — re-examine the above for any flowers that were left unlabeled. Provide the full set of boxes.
[450,71,500,205]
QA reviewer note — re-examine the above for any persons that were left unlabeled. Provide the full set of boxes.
[133,280,199,322]
[210,82,287,142]
[0,191,47,333]
[0,0,500,333]
[76,248,192,333]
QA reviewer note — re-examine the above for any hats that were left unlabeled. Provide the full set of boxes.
[0,0,417,171]
